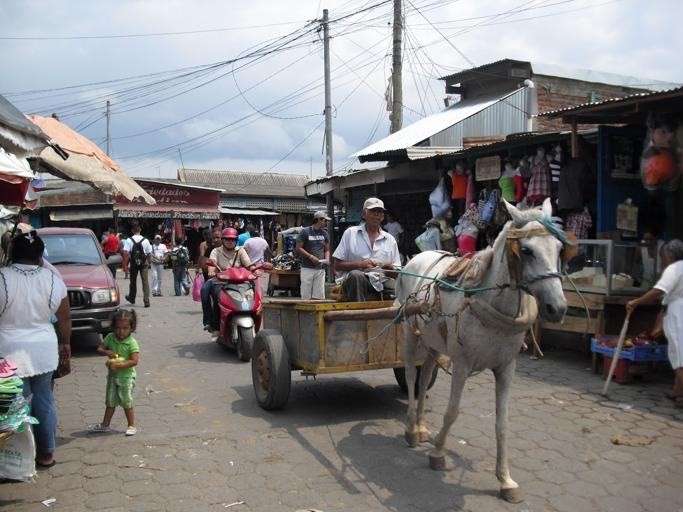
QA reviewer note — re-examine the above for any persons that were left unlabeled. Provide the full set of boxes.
[413,143,590,269]
[380,211,404,248]
[0,229,73,470]
[85,304,140,438]
[332,196,401,301]
[630,228,669,291]
[625,238,683,409]
[0,219,277,334]
[294,209,330,302]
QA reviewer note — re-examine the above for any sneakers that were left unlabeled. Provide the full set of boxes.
[88,424,111,432]
[125,425,136,435]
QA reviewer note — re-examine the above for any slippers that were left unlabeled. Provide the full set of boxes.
[0,357,25,413]
[36,458,57,468]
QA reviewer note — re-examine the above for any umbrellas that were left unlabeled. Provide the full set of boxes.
[5,115,157,239]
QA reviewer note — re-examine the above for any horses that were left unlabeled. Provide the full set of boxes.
[393,195,569,504]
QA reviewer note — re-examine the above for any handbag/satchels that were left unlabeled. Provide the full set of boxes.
[192,272,203,301]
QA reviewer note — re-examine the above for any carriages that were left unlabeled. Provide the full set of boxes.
[250,198,576,504]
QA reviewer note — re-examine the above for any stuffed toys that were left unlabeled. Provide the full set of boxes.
[640,108,681,193]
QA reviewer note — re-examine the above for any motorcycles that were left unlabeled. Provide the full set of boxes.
[196,256,276,365]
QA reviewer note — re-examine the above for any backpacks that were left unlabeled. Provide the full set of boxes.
[172,245,189,265]
[129,237,148,267]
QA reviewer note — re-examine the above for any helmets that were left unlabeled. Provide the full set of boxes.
[221,228,239,239]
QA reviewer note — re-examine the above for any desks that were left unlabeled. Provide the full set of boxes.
[265,270,301,298]
[532,290,665,374]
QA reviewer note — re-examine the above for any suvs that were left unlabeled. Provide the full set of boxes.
[32,225,124,344]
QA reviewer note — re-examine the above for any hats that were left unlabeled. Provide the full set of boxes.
[363,197,386,212]
[155,234,162,240]
[314,211,332,220]
